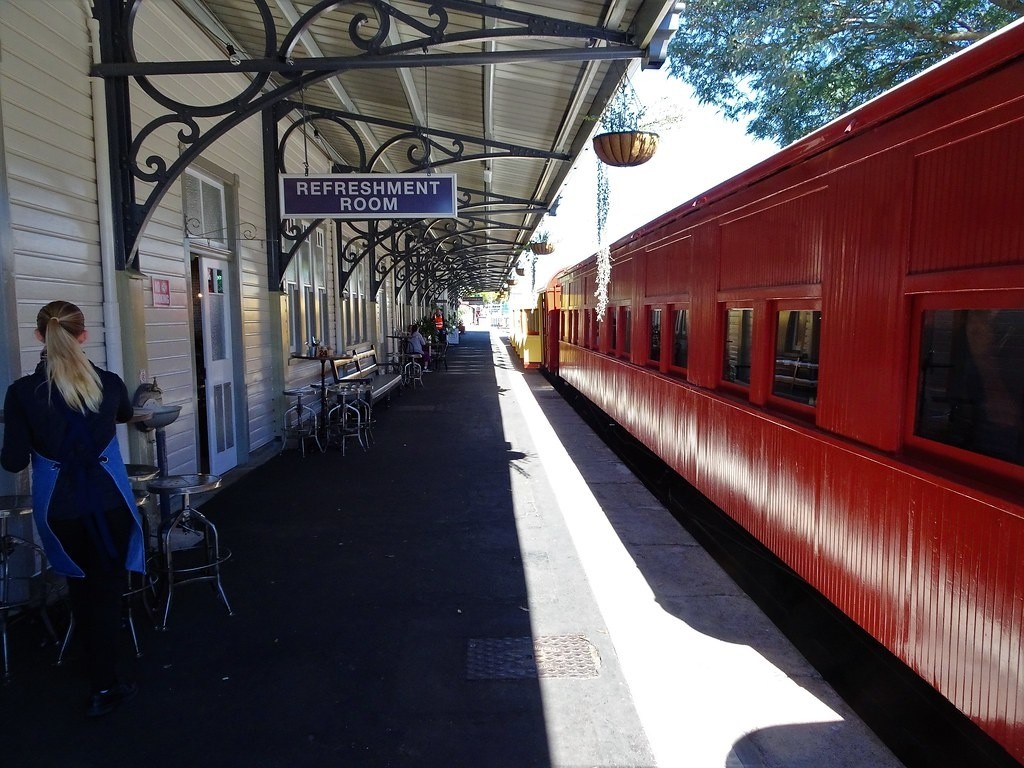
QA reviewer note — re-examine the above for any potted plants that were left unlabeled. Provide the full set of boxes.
[498,230,555,298]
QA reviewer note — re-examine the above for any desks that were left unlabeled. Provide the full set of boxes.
[292,353,353,453]
[387,334,415,352]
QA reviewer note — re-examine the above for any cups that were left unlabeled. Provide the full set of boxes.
[317,348,327,356]
[309,347,316,358]
[328,350,333,356]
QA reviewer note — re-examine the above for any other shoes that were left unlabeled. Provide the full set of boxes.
[422,367,434,372]
[84,682,139,718]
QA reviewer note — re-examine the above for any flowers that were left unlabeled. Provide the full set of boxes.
[582,94,685,132]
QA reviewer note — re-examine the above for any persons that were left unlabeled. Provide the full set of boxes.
[406,325,433,372]
[0,302,146,717]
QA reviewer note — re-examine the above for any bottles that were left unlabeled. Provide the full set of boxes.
[395,329,402,337]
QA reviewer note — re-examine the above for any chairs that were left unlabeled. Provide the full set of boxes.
[432,340,449,373]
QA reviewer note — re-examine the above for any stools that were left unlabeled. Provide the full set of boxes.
[385,351,424,391]
[278,381,375,460]
[0,494,61,688]
[146,472,237,632]
[124,463,161,660]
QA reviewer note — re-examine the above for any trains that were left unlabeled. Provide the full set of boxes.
[501,14,1024,768]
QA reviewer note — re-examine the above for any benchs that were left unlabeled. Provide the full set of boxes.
[330,344,406,421]
